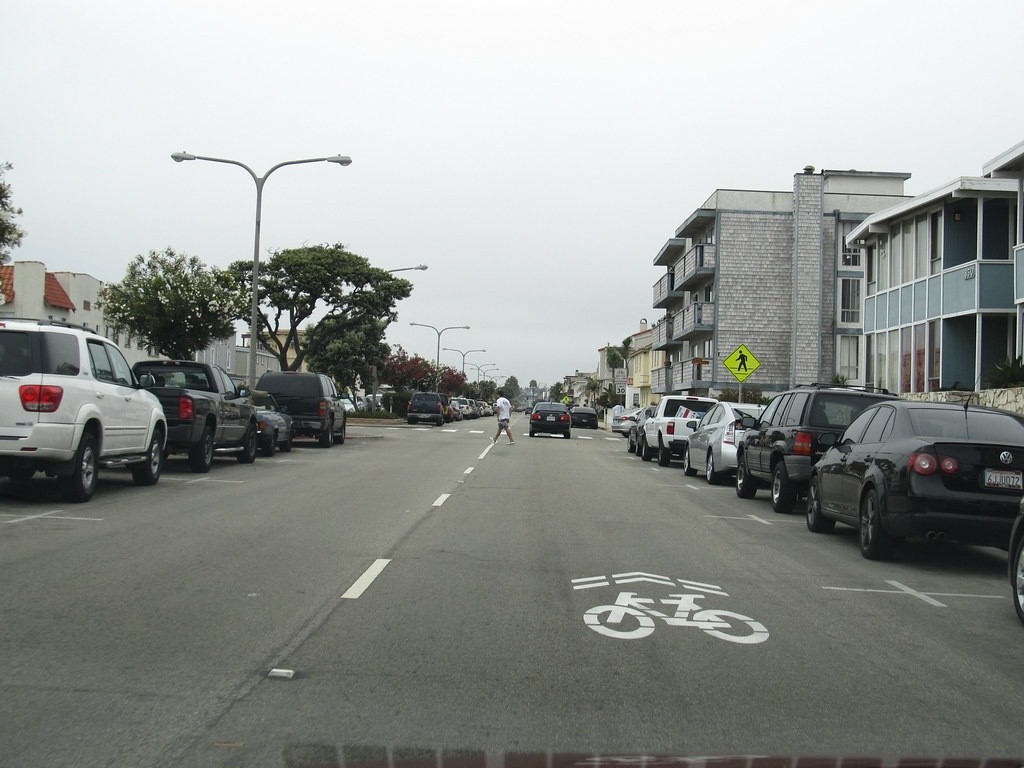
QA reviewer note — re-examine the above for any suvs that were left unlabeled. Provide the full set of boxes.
[736,383,906,513]
[1,317,168,503]
[406,392,495,426]
[255,370,347,448]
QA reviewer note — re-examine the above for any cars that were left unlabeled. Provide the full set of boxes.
[612,406,656,456]
[529,402,572,439]
[684,402,768,484]
[237,390,294,457]
[340,394,386,413]
[566,406,598,429]
[806,400,1024,560]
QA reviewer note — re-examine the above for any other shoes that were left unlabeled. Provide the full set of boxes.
[506,441,515,445]
[490,437,497,444]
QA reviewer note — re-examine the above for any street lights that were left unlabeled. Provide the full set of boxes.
[442,347,486,379]
[409,322,471,394]
[462,362,507,385]
[170,151,353,395]
[370,264,428,415]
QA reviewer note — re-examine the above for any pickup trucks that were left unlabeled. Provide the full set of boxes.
[642,395,718,467]
[130,360,258,472]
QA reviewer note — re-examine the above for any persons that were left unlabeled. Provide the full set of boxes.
[490,390,515,444]
[612,402,625,415]
